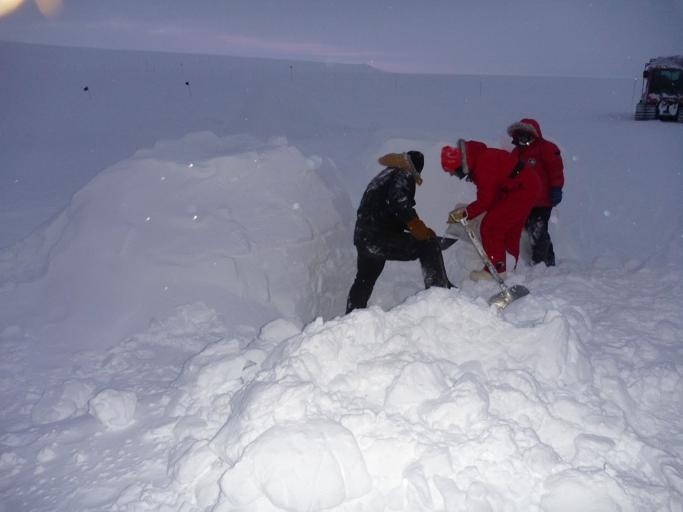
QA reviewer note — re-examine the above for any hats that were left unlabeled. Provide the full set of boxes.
[441,147,461,172]
[407,150,424,173]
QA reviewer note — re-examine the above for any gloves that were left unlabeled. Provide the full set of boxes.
[449,208,465,224]
[410,218,435,240]
[550,188,562,206]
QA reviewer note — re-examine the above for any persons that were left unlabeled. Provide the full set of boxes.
[441,138,543,282]
[345,150,458,315]
[508,119,565,268]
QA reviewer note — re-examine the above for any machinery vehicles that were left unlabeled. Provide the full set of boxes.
[634,55,683,124]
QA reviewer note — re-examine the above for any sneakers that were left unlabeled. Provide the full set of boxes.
[471,270,505,280]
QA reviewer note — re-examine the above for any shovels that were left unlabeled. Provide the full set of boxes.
[450,205,529,310]
